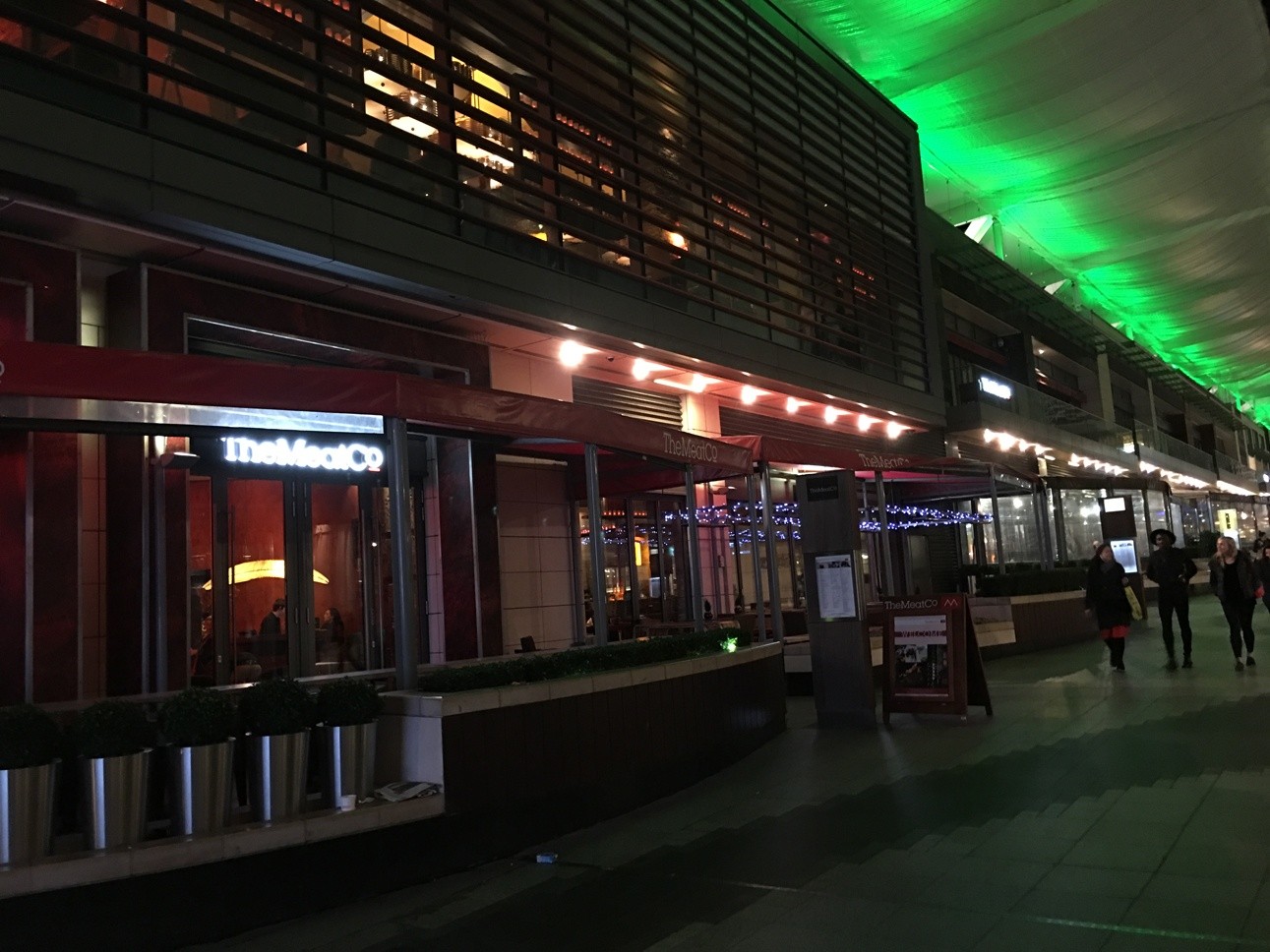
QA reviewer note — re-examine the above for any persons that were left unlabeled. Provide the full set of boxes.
[1083,542,1132,673]
[1207,537,1260,670]
[259,597,286,662]
[1145,528,1197,670]
[320,608,346,654]
[201,612,216,662]
[1251,531,1270,614]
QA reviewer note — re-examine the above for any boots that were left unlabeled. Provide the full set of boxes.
[1114,637,1125,670]
[1105,637,1116,668]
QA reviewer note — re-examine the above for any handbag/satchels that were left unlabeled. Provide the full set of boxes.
[1124,586,1143,622]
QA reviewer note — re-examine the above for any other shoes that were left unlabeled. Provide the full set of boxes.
[1235,662,1244,671]
[1246,656,1255,666]
[1167,659,1177,670]
[1182,656,1193,667]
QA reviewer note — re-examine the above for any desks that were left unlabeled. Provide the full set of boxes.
[235,630,328,668]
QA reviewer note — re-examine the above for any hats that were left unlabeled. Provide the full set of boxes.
[1149,528,1177,546]
